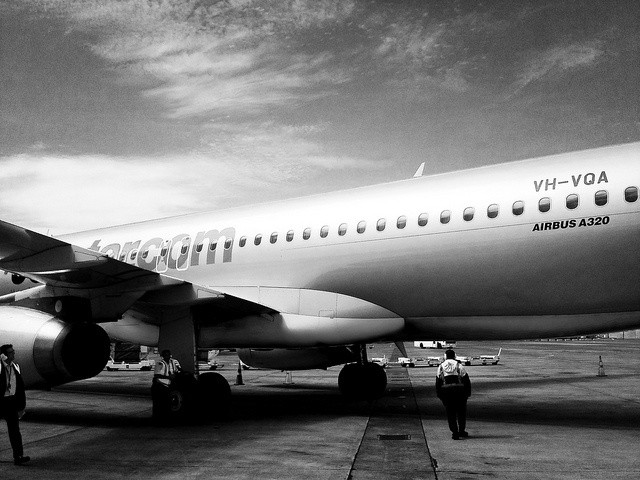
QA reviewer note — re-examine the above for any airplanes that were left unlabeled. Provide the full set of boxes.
[0,143,640,424]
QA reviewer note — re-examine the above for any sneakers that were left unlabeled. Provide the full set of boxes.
[461,431,468,437]
[15,456,30,463]
[452,433,461,439]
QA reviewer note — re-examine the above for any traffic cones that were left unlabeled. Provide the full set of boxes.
[235,362,244,385]
[596,355,607,377]
[285,370,291,385]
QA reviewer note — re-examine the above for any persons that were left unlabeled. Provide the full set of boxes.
[0,343,29,466]
[433,348,472,441]
[150,349,182,423]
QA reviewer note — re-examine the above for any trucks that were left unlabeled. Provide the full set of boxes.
[413,340,457,349]
